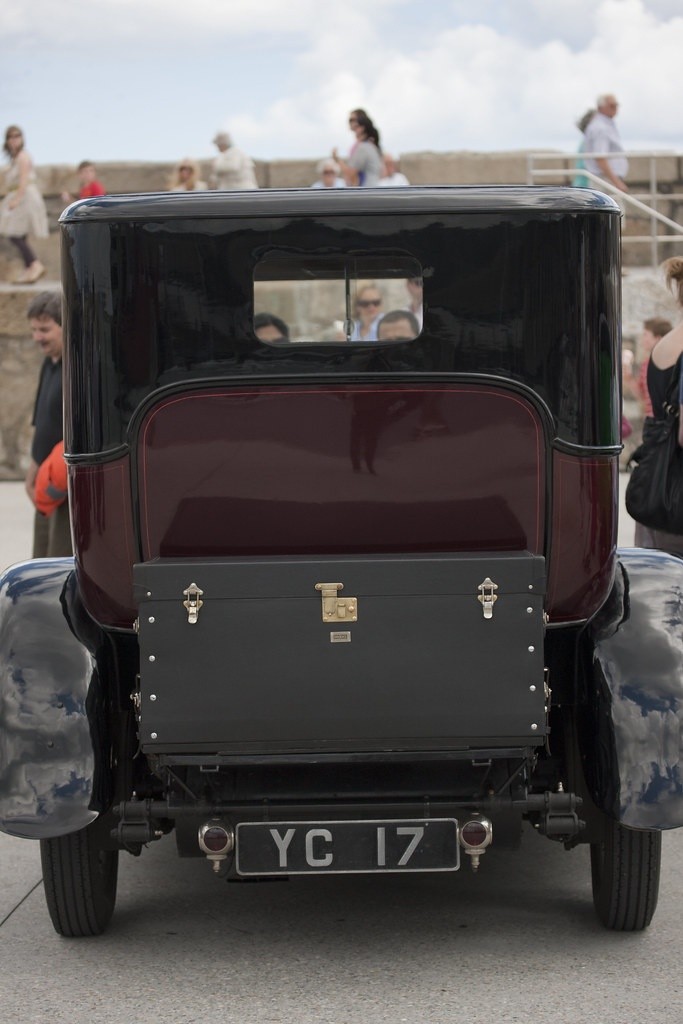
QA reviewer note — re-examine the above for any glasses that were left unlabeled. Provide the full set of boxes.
[7,133,21,138]
[357,299,381,308]
[349,118,358,123]
[324,170,335,175]
[180,166,193,172]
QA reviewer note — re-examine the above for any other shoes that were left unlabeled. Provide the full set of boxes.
[12,259,45,283]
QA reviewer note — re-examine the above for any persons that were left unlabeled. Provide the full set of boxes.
[22,289,73,559]
[572,92,633,232]
[209,131,259,192]
[308,107,411,190]
[0,124,50,284]
[252,276,424,347]
[625,257,683,560]
[168,157,209,193]
[61,155,107,206]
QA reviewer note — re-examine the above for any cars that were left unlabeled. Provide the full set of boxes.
[2,188,683,940]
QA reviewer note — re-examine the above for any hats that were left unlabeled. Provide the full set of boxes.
[213,132,233,144]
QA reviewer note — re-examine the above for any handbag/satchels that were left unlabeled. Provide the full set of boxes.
[624,397,683,535]
